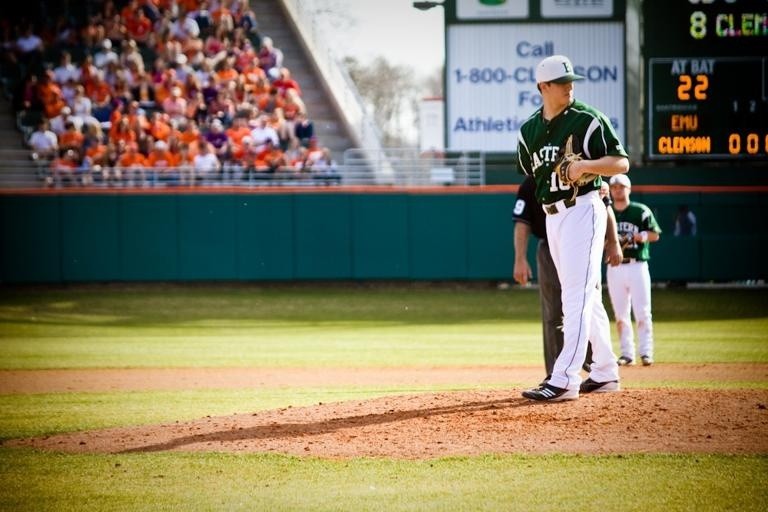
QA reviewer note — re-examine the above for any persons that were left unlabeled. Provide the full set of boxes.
[510,54,632,402]
[607,174,666,370]
[1,0,342,186]
[513,139,594,381]
[596,182,613,214]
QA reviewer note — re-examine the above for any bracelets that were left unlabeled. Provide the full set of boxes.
[641,231,649,243]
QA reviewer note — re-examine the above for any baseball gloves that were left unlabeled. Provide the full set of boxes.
[555,152,599,201]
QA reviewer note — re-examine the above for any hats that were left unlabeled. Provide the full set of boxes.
[535,55,585,83]
[608,174,631,190]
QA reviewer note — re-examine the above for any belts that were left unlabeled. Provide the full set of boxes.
[545,198,576,214]
[622,258,639,264]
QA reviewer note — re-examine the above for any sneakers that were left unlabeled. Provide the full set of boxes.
[522,379,620,402]
[617,354,653,366]
[582,363,591,373]
